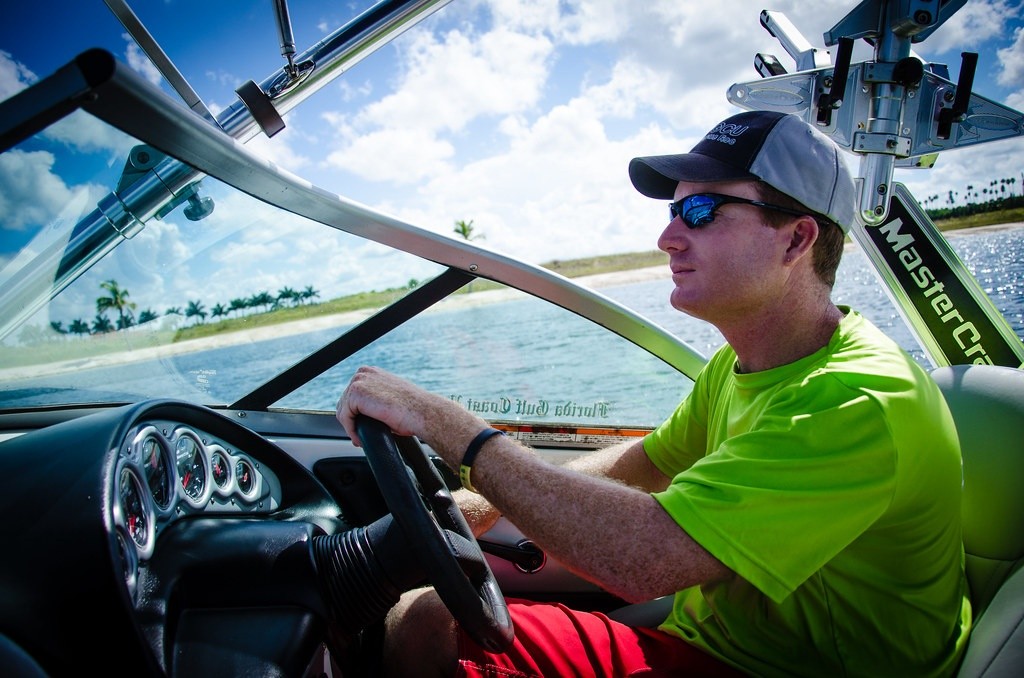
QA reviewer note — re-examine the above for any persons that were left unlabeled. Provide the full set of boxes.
[335,111,974,678]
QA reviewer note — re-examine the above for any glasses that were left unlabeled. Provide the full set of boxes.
[669,191,830,230]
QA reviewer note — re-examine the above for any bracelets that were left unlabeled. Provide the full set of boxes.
[459,428,506,494]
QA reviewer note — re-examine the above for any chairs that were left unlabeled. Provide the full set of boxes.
[926,364,1023,678]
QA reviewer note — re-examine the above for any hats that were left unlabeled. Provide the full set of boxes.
[628,110,855,235]
